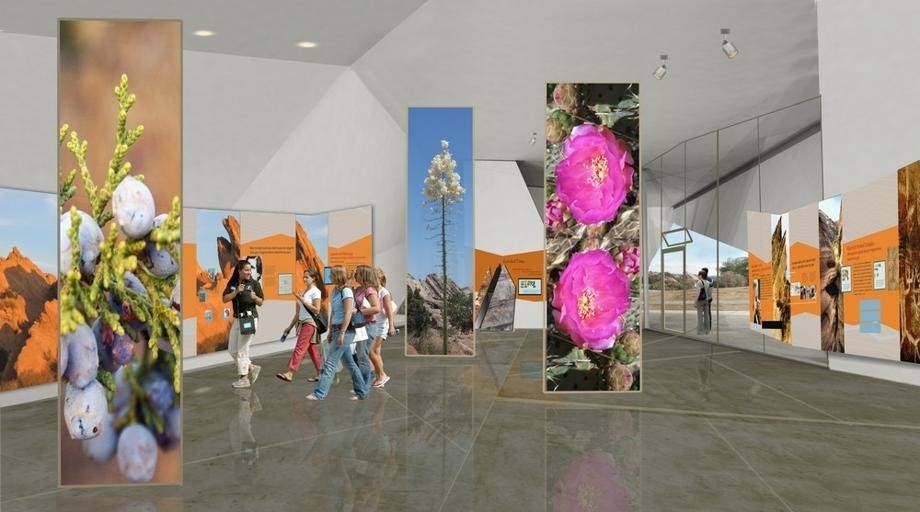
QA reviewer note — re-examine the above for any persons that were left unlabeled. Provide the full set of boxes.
[223,260,264,388]
[305,265,369,401]
[368,268,397,388]
[276,270,329,383]
[228,389,263,470]
[350,265,381,395]
[322,388,397,512]
[693,270,710,335]
[702,268,714,330]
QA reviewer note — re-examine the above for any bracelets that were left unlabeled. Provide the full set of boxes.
[339,330,345,334]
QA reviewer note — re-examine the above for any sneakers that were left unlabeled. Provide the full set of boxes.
[230,365,262,389]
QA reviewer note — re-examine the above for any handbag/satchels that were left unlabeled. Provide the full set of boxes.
[352,312,365,326]
[314,311,329,332]
[240,315,255,334]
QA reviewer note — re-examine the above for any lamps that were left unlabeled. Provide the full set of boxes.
[653,54,668,79]
[720,27,740,59]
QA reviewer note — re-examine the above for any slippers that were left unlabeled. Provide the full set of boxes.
[371,375,390,387]
[277,370,294,382]
[307,375,320,382]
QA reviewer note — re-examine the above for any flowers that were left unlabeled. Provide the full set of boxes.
[547,82,643,393]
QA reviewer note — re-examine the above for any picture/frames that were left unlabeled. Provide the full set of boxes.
[872,260,886,291]
[517,278,542,296]
[839,264,852,293]
